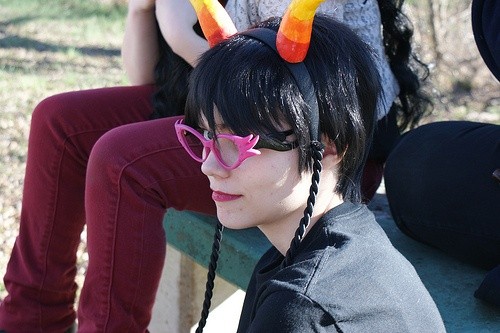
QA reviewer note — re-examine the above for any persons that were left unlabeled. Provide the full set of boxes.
[0,0,434,333]
[185,13,447,333]
[384,0,500,308]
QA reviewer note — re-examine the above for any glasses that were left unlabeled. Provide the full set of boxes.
[174,117,302,170]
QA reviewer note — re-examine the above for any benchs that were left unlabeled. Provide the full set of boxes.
[146,193,500,333]
